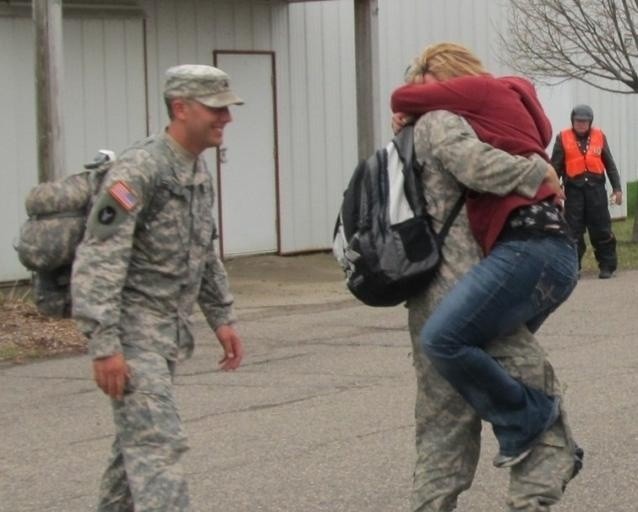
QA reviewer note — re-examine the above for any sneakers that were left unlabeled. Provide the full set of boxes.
[599,269,611,278]
[491,445,534,468]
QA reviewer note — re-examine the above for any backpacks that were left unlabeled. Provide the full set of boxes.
[334,109,466,306]
[17,146,170,317]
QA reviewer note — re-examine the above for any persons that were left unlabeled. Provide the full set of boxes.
[388,109,586,512]
[386,39,582,470]
[66,59,248,512]
[549,100,626,282]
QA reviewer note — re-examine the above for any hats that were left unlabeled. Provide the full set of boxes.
[165,65,244,107]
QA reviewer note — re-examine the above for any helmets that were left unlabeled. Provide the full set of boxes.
[571,105,593,128]
